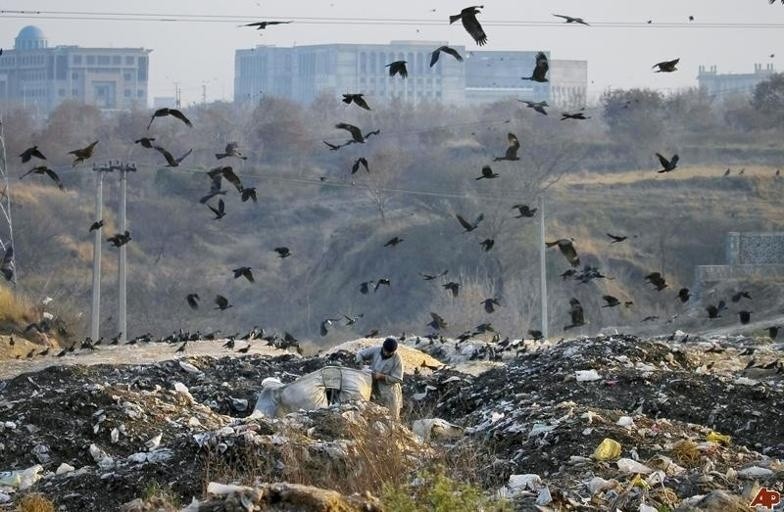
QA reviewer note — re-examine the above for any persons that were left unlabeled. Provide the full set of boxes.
[2,246,15,280]
[355,338,403,420]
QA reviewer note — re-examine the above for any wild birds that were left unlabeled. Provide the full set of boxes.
[7,285,783,377]
[231,266,256,284]
[240,18,291,32]
[18,107,258,249]
[655,151,680,175]
[551,13,587,27]
[652,57,682,76]
[318,91,379,183]
[0,245,13,284]
[516,51,591,120]
[384,4,490,79]
[272,244,290,258]
[476,132,522,182]
[357,203,669,297]
